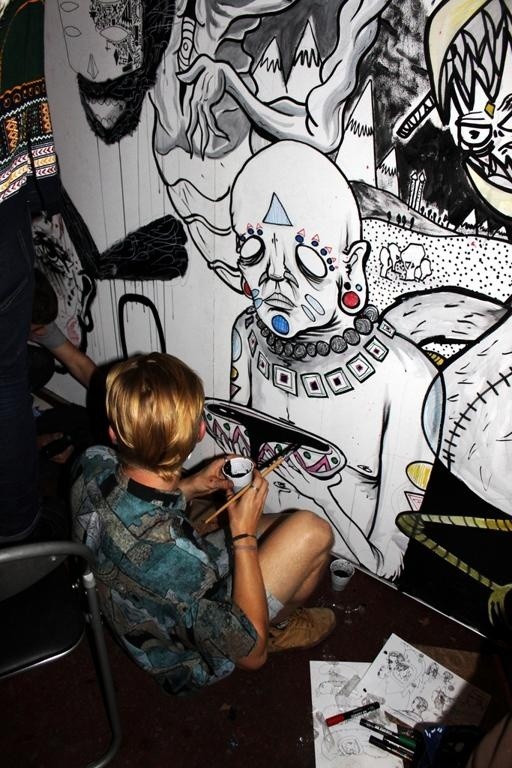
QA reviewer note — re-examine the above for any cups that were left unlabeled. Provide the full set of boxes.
[221,457,255,497]
[330,559,354,592]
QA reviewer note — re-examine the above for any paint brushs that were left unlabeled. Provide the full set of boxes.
[204,443,302,524]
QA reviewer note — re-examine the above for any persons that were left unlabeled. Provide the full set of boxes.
[0,184,46,548]
[1,268,100,466]
[69,351,337,697]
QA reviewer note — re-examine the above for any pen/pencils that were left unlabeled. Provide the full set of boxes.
[326,702,417,762]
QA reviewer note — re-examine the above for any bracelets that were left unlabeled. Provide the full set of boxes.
[230,533,257,543]
[231,544,257,552]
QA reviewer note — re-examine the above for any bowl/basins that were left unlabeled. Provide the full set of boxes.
[199,396,348,479]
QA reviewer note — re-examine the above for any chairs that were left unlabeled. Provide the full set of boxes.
[1,493,122,766]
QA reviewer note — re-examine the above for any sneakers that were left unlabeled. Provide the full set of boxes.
[266,606,337,654]
